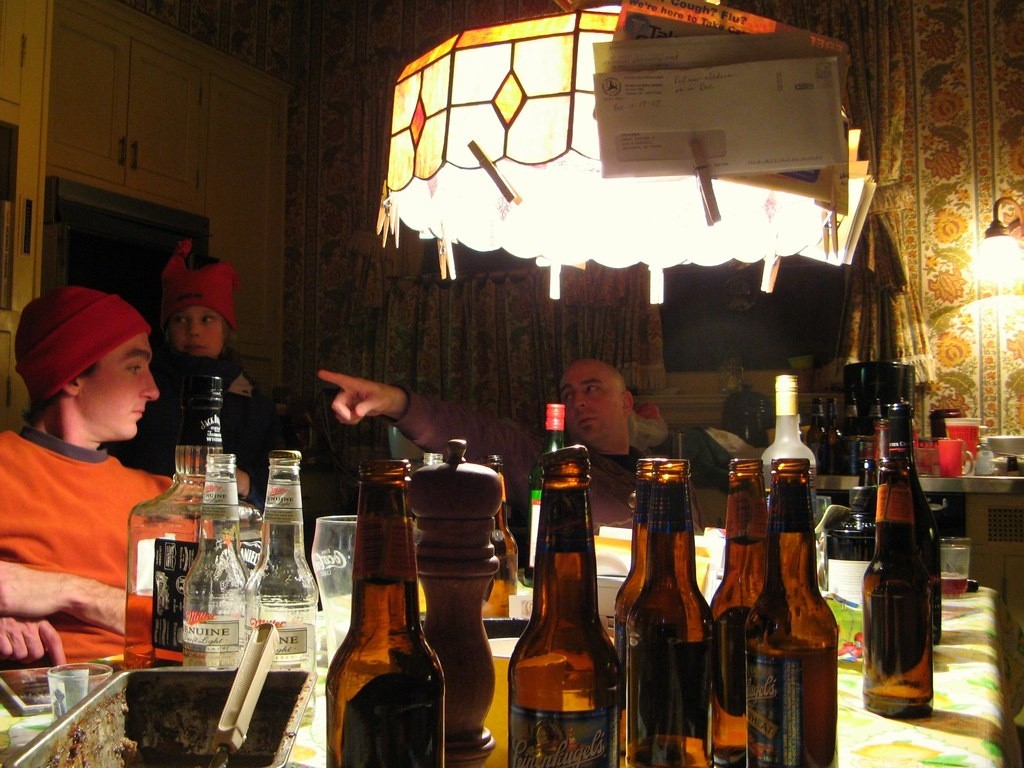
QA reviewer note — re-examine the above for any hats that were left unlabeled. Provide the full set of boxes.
[159,241,239,334]
[15,287,151,410]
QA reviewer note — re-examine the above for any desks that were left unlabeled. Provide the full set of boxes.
[0,574,1002,768]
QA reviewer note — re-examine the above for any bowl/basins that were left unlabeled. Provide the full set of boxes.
[987,436,1023,456]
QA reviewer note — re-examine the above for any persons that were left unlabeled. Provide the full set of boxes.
[0,286,273,669]
[119,240,290,509]
[317,358,706,546]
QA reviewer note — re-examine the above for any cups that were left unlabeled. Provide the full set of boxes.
[311,516,358,667]
[944,417,982,461]
[939,537,971,596]
[47,662,114,724]
[938,439,974,477]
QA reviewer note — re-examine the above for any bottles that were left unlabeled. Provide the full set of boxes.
[612,457,658,767]
[523,404,567,587]
[719,383,769,448]
[244,450,318,667]
[745,459,838,768]
[123,373,264,672]
[325,457,446,768]
[759,374,944,720]
[711,459,768,767]
[182,454,252,666]
[975,438,995,475]
[417,452,519,614]
[507,445,618,767]
[625,458,710,768]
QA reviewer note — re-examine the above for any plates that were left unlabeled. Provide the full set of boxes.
[989,456,1024,471]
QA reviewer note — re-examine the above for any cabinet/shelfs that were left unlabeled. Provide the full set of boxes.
[0,0,284,437]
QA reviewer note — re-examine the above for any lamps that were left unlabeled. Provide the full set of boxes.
[975,195,1024,275]
[376,0,848,304]
